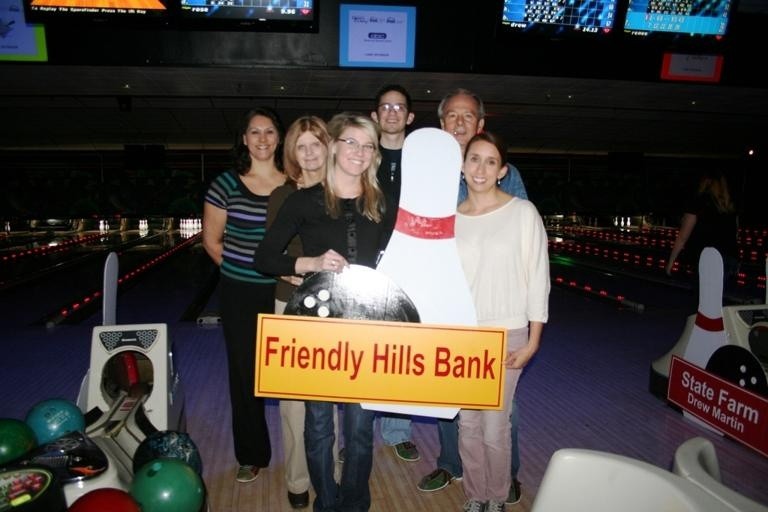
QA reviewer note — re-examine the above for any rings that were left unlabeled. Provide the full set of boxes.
[331,259,337,267]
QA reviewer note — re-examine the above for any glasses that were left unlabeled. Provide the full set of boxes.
[374,102,409,113]
[332,136,377,155]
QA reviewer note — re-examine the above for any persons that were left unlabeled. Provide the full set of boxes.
[250,111,395,512]
[416,85,532,506]
[662,166,743,310]
[201,105,287,484]
[261,113,339,512]
[457,125,552,512]
[370,84,424,461]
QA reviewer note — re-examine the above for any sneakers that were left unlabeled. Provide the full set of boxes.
[464,499,485,512]
[415,467,462,493]
[505,477,525,505]
[234,465,261,483]
[485,499,504,512]
[338,449,348,463]
[394,439,422,462]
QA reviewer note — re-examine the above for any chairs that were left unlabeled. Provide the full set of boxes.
[670,436,765,510]
[528,445,732,511]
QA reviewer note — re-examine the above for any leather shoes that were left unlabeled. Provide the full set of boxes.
[334,483,343,502]
[287,490,311,509]
